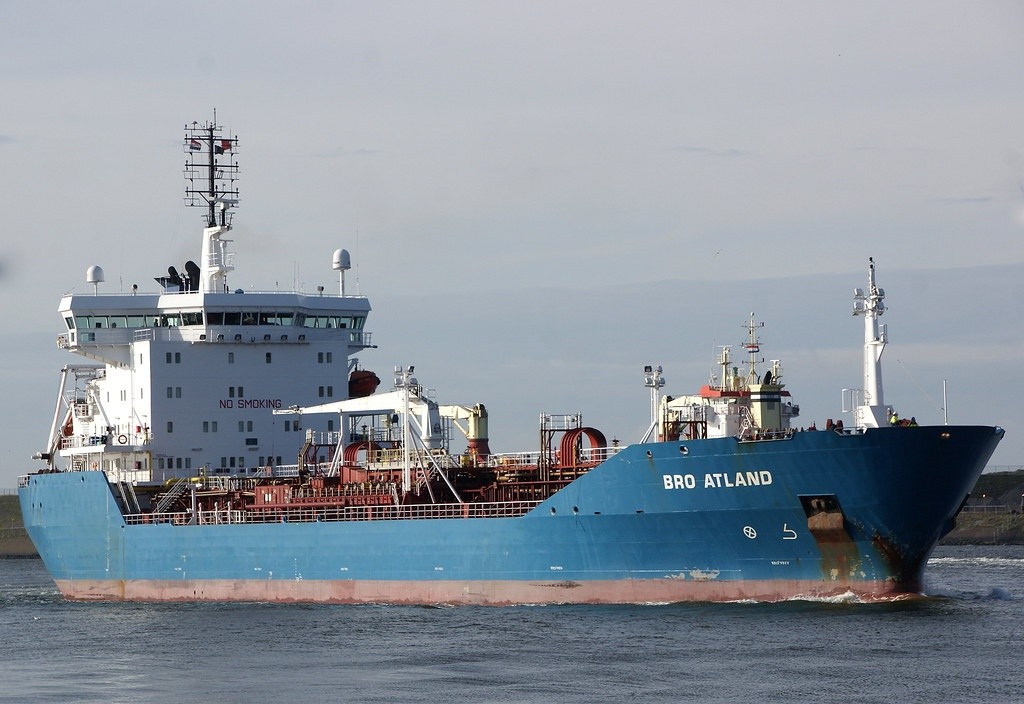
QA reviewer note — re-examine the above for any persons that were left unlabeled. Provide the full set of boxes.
[890,411,899,427]
[908,417,918,426]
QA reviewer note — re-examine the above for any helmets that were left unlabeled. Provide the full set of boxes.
[892,411,898,415]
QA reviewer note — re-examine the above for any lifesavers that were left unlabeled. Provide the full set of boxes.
[118,435,127,444]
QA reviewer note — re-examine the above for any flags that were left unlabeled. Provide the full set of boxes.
[215,145,224,155]
[222,141,230,150]
[190,140,201,150]
[745,346,760,353]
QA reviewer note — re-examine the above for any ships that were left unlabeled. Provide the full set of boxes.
[18,104,1005,603]
